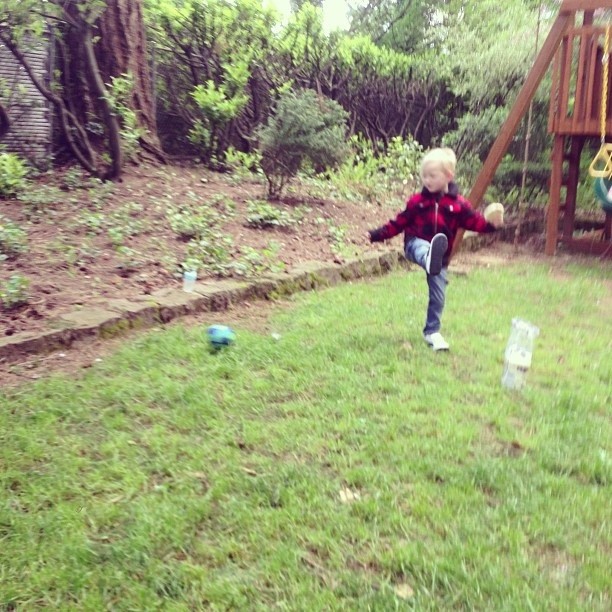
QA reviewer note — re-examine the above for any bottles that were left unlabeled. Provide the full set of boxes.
[182,268,197,293]
[501,319,538,388]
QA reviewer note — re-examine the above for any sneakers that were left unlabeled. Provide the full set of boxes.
[425,233,448,275]
[425,333,448,350]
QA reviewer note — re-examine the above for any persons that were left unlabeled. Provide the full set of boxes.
[363,147,509,353]
